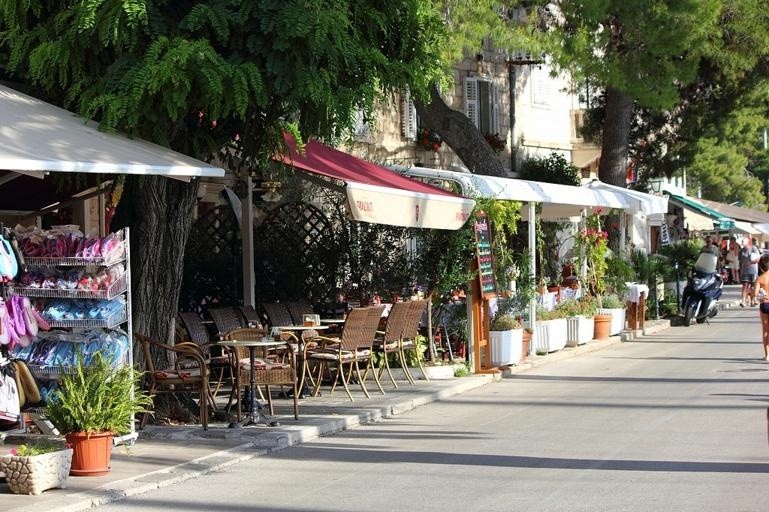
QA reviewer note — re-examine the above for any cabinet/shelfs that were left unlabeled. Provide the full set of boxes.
[2,222,139,450]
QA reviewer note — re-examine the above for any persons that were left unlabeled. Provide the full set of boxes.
[700,236,763,307]
[750,255,769,361]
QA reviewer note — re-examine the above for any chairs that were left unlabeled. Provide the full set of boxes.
[136,333,210,431]
[224,329,299,420]
[379,300,428,381]
[363,302,413,388]
[309,308,371,402]
[179,313,239,397]
[330,305,385,399]
[208,301,314,337]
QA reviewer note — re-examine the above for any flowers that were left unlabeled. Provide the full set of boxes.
[483,131,509,152]
[415,124,444,154]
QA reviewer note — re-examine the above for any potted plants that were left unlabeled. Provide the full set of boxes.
[0,437,74,496]
[432,235,689,366]
[36,351,157,475]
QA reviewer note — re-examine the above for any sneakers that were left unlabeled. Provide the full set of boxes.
[12,336,127,368]
[42,382,65,402]
[23,231,120,262]
[0,294,51,350]
[20,263,124,296]
[0,234,17,284]
[43,297,127,319]
[12,359,41,408]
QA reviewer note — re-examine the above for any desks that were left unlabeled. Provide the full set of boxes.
[217,340,280,429]
[272,326,321,398]
[320,319,346,322]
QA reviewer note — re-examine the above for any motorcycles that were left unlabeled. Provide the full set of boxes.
[681,250,724,326]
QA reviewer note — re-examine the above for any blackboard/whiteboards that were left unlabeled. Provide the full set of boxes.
[473,210,498,299]
